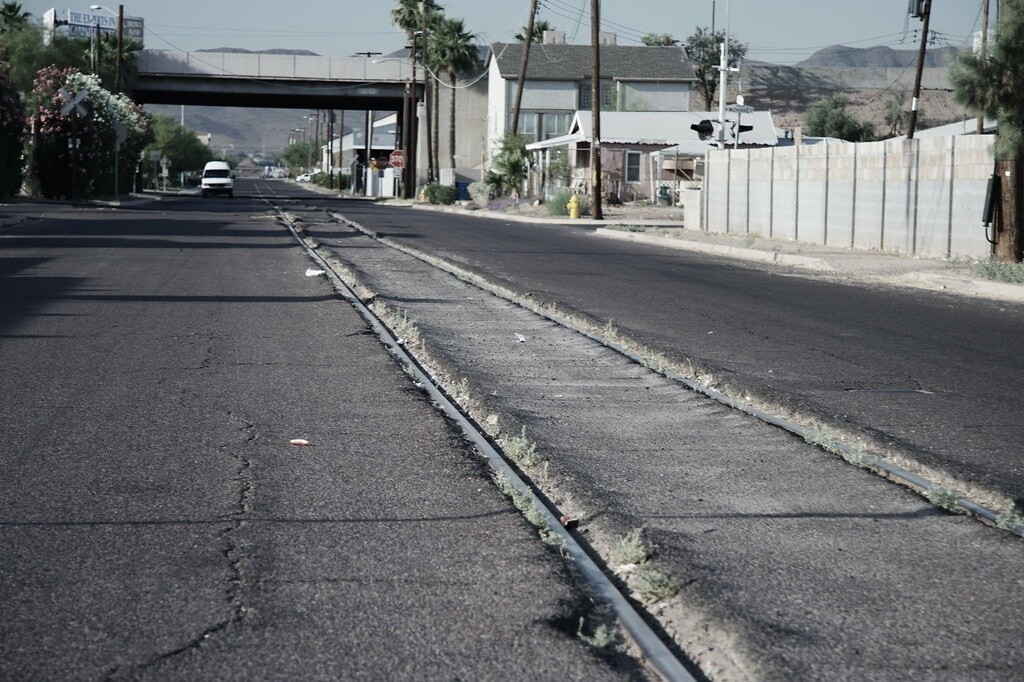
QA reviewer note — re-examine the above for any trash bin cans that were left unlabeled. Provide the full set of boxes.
[684,187,703,230]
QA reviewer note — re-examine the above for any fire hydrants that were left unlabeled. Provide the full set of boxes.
[566,195,579,218]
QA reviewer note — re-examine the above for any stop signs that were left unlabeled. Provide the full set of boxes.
[390,150,403,168]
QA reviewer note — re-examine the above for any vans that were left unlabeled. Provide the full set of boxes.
[200,161,235,198]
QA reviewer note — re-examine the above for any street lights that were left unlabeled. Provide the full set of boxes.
[89,4,124,199]
